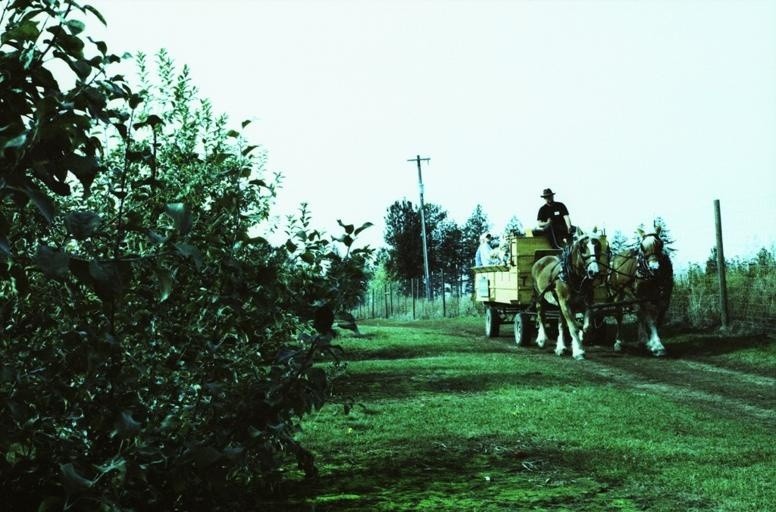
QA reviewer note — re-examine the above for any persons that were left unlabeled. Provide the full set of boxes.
[536,188,573,250]
[475,227,523,268]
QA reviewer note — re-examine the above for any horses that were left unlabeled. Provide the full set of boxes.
[604,225,669,357]
[530,224,602,361]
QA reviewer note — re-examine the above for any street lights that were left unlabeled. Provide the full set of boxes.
[406,157,434,303]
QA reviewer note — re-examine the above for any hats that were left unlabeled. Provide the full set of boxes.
[541,188,556,198]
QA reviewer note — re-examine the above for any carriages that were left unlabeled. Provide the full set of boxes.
[472,229,675,360]
